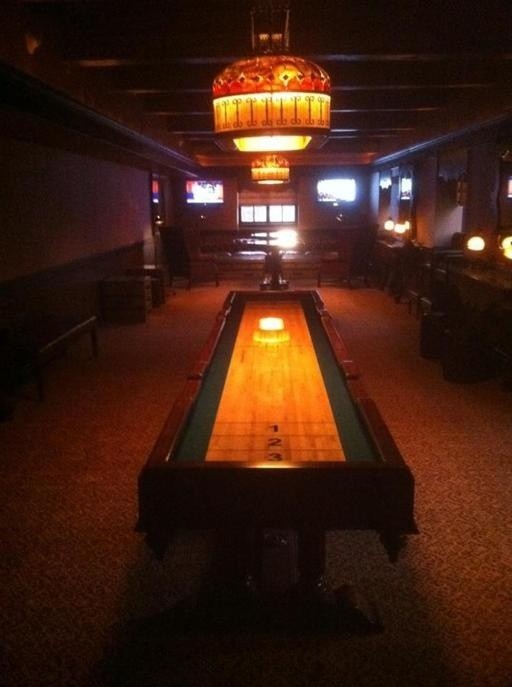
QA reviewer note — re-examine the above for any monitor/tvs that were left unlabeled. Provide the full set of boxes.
[315,176,362,206]
[184,177,226,207]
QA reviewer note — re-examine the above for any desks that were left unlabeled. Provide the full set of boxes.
[133,290,420,637]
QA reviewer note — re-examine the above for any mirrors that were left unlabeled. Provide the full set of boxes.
[398,165,416,239]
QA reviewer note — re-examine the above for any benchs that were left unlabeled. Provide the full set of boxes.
[198,252,339,288]
[21,313,99,398]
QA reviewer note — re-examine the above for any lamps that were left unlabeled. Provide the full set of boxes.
[212,0,333,140]
[250,155,291,185]
